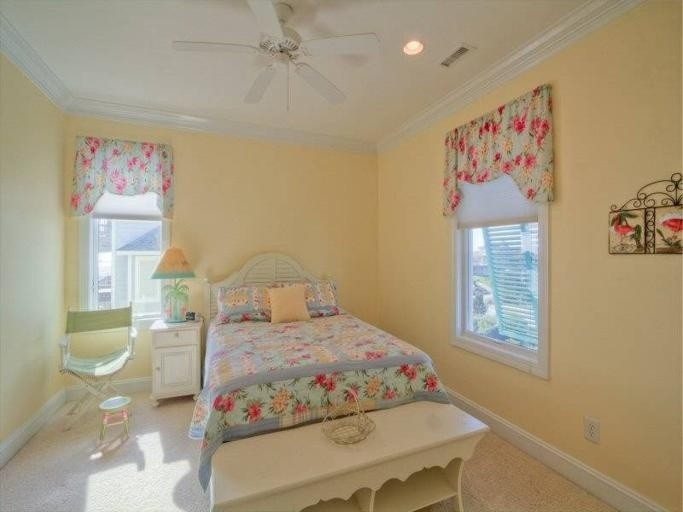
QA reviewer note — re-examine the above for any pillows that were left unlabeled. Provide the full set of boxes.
[214,280,338,326]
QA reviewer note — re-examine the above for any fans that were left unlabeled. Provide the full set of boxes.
[169,1,381,111]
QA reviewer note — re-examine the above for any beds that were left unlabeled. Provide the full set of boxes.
[200,251,435,423]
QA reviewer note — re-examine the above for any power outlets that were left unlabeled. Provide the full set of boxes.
[582,416,602,443]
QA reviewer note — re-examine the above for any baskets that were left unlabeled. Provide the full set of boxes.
[321,387,376,445]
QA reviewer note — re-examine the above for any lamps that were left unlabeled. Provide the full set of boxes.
[147,246,198,323]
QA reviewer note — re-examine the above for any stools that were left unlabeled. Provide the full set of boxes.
[94,394,135,446]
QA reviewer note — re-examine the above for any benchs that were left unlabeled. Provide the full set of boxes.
[208,398,490,511]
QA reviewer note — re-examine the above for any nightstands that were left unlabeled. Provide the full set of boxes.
[149,317,203,409]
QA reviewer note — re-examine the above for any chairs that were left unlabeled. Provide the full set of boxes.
[57,302,142,431]
